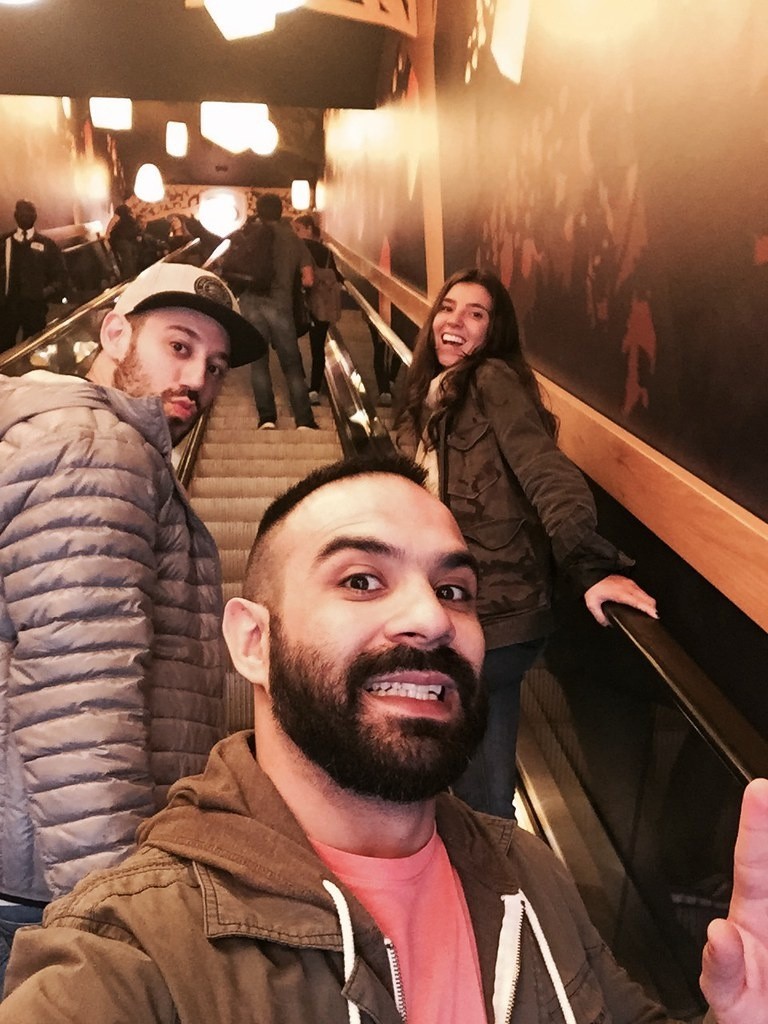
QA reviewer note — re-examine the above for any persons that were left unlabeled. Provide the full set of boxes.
[221,192,321,430]
[387,267,659,823]
[281,215,350,406]
[108,205,203,281]
[1,261,263,1006]
[0,199,70,354]
[2,453,767,1024]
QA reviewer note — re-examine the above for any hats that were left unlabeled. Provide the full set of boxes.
[112,262,267,369]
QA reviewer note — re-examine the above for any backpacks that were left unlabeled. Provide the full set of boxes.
[303,248,342,323]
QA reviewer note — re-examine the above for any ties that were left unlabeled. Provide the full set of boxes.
[22,231,28,244]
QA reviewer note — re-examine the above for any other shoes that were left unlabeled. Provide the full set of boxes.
[258,420,276,430]
[296,422,321,431]
[309,391,321,405]
